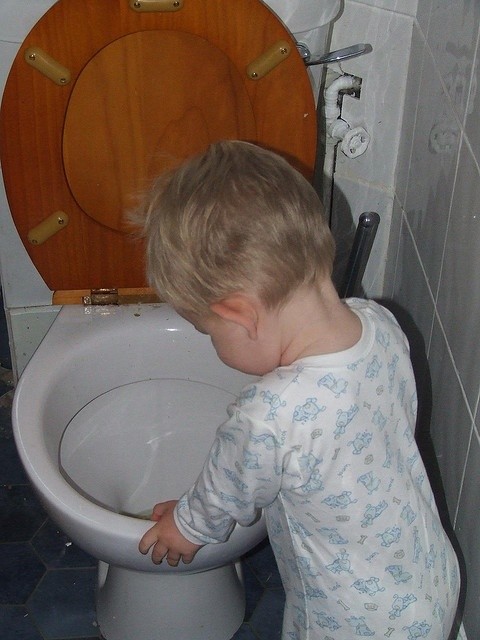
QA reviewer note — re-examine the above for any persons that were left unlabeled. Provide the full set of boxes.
[137,140,462,640]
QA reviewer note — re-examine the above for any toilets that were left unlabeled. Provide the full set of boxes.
[2,1,346,640]
